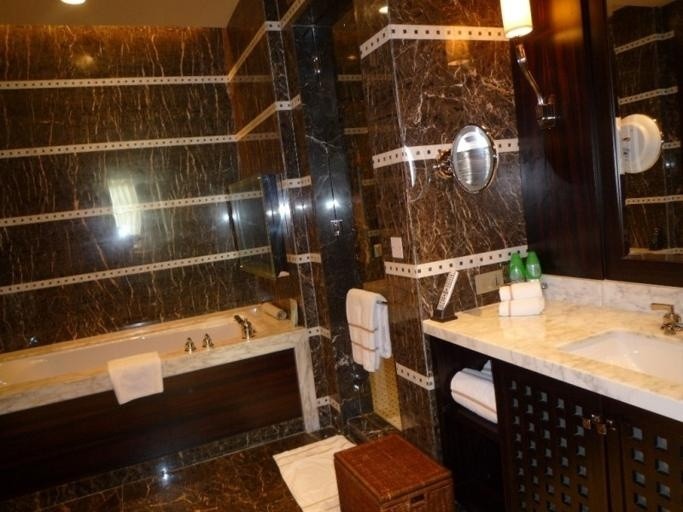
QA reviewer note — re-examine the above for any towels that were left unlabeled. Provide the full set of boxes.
[105,350,164,409]
[496,279,547,318]
[342,286,393,373]
[446,359,495,425]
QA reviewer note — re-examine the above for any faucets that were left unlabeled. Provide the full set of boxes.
[650,300,682,335]
[233,314,255,341]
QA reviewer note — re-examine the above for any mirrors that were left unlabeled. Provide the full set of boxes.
[430,123,498,197]
[582,0,682,286]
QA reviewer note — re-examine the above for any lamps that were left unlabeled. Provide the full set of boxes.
[495,1,558,130]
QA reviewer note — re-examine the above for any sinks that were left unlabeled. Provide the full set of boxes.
[555,324,683,389]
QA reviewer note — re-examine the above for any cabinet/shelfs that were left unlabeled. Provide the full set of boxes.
[428,336,682,511]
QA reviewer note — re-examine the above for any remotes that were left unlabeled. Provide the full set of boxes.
[436,270,459,310]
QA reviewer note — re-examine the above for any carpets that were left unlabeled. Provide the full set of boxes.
[271,433,359,512]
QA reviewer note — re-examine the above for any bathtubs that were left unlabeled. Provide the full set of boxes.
[0,301,307,416]
